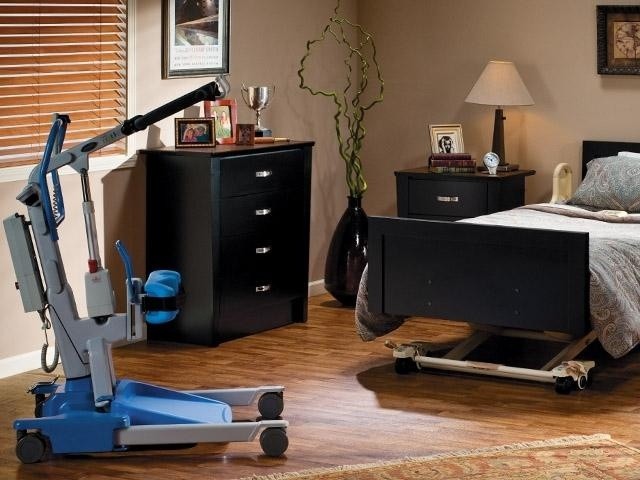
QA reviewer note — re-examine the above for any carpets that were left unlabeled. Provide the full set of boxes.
[242,433,638,480]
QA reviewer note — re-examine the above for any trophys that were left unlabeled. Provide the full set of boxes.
[240,80,277,137]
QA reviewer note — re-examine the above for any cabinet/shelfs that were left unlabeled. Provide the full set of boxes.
[136,139,316,348]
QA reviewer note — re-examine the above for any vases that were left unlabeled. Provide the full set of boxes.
[324,195,370,307]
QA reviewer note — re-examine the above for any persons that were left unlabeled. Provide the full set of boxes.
[183,127,198,142]
[193,126,209,142]
[438,135,455,154]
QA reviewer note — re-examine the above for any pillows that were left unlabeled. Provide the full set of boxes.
[568,151,640,210]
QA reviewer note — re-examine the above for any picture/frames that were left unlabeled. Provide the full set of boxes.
[596,5,640,75]
[161,1,231,79]
[173,100,254,149]
[428,124,465,153]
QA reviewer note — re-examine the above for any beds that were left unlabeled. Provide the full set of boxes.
[369,141,640,396]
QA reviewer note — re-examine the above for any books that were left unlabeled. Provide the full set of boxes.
[428,166,477,173]
[431,152,471,160]
[429,159,477,168]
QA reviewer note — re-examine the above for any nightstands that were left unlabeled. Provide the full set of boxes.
[393,166,535,222]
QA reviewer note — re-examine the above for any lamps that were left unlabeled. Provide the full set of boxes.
[464,60,536,171]
